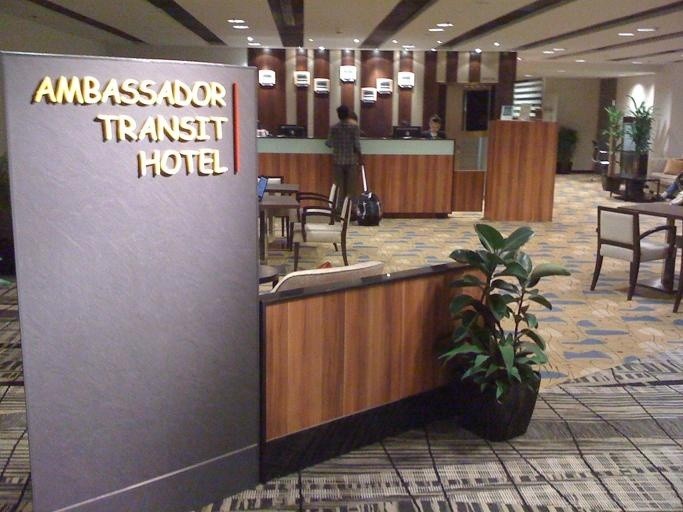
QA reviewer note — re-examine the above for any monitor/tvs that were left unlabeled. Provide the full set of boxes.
[391,125,422,138]
[278,124,306,139]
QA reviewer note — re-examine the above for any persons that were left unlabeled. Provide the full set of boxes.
[349,113,369,136]
[421,115,447,140]
[325,106,364,223]
[656,171,683,206]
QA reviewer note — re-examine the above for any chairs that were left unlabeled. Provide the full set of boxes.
[591,206,677,300]
[258,176,384,294]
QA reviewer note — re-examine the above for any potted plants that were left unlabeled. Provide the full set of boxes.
[601,104,621,191]
[432,223,571,441]
[625,95,656,185]
[556,127,578,173]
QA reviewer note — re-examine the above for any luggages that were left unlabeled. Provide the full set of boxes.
[355,163,382,225]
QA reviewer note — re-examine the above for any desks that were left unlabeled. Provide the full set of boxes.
[617,204,683,293]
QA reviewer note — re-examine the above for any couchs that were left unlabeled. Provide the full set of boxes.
[647,157,683,194]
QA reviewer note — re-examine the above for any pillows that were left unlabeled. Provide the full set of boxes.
[664,158,683,175]
